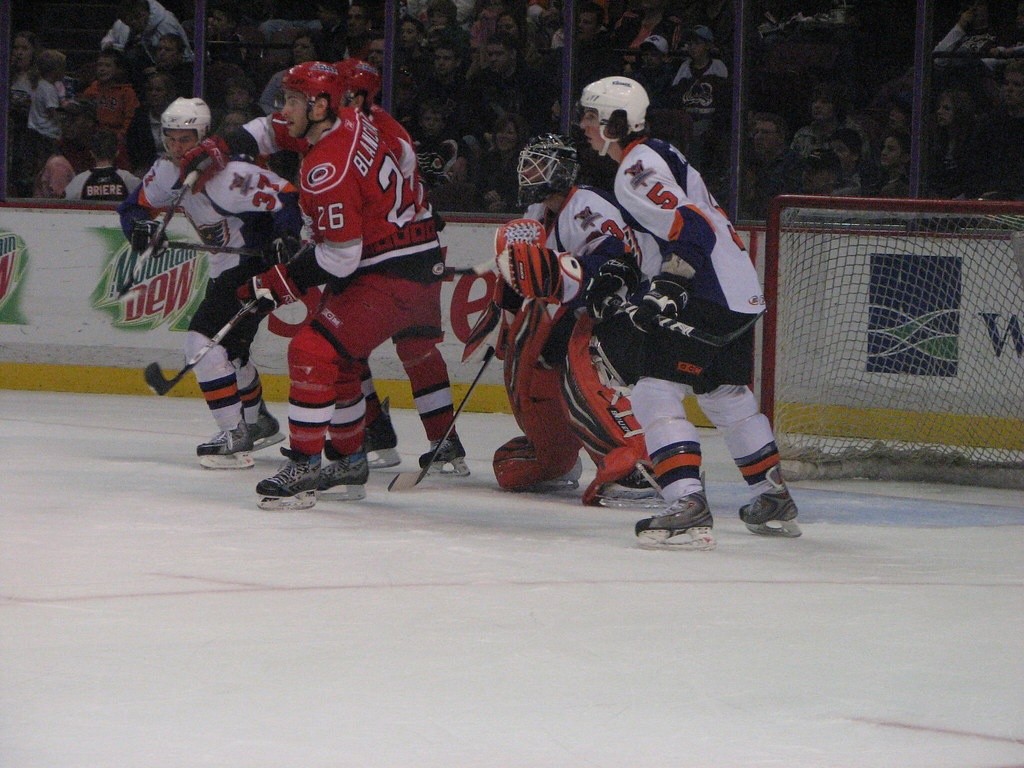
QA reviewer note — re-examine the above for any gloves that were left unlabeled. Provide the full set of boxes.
[129,218,170,258]
[589,259,638,318]
[236,266,308,314]
[633,274,689,333]
[180,134,232,195]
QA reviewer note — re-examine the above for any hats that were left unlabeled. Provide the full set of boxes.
[684,26,713,42]
[637,35,668,53]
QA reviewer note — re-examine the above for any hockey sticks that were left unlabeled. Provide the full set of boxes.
[143,302,253,396]
[117,158,204,305]
[387,353,495,490]
[601,287,768,351]
[156,229,497,281]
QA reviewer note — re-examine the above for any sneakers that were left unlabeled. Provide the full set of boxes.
[601,467,660,508]
[256,447,325,510]
[364,398,403,468]
[739,464,802,536]
[514,458,585,493]
[419,434,471,476]
[239,400,287,452]
[195,409,255,468]
[315,442,370,500]
[635,472,715,551]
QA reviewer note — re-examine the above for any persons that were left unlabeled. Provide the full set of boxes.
[495,77,801,549]
[0,0,1024,214]
[118,97,299,469]
[254,63,427,509]
[335,58,469,476]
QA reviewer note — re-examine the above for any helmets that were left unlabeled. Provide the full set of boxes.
[336,59,381,102]
[580,76,650,143]
[515,133,582,206]
[159,98,211,159]
[282,61,343,113]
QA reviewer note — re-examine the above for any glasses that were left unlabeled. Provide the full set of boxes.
[165,135,198,146]
